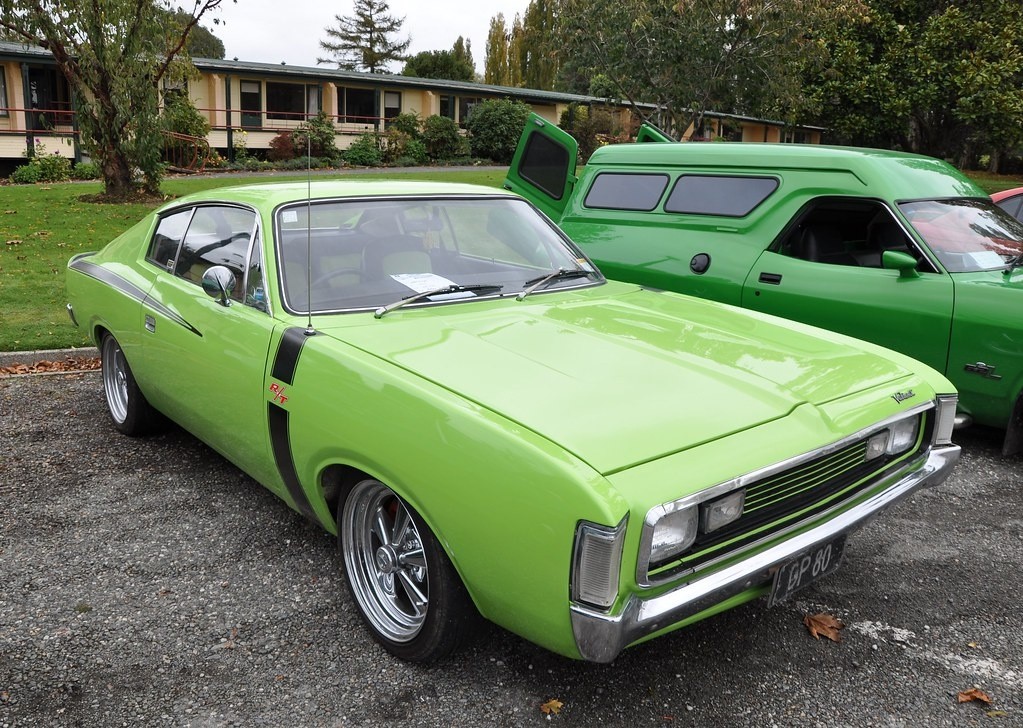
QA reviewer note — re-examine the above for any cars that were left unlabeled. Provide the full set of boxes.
[68,176,962,667]
[503,111,1022,462]
[911,186,1023,265]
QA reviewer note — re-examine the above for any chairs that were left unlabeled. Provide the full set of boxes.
[176,236,434,298]
[791,218,884,269]
